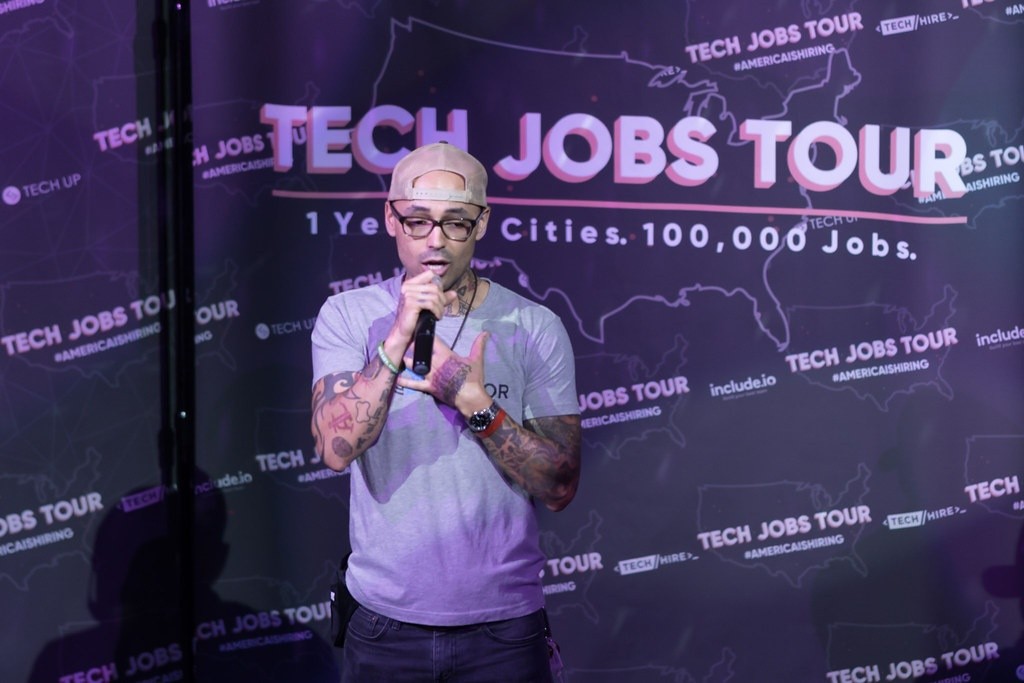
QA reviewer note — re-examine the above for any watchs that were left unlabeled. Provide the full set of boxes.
[465,402,501,433]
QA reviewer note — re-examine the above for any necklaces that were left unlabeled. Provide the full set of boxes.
[450,267,477,350]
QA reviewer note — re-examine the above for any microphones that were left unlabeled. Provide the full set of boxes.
[411,273,441,376]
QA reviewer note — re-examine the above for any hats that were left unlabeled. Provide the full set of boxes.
[387,141,487,207]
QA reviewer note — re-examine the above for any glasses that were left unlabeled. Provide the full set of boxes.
[390,204,485,242]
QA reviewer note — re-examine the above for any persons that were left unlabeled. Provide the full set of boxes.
[311,140,580,683]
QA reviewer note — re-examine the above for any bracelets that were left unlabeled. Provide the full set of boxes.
[476,409,506,439]
[378,341,399,374]
